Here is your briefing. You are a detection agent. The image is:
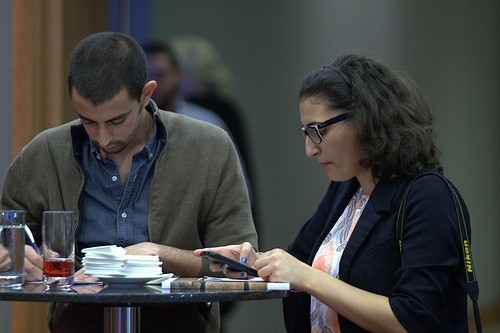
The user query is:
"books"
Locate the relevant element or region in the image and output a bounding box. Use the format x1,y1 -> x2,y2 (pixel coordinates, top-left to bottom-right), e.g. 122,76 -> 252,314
162,278 -> 291,291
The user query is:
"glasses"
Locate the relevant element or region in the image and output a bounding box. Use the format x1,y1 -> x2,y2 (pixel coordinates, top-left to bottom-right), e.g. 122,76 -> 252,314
298,111 -> 355,145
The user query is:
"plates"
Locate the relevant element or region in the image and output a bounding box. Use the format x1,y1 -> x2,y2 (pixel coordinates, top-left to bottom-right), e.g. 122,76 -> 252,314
95,277 -> 154,287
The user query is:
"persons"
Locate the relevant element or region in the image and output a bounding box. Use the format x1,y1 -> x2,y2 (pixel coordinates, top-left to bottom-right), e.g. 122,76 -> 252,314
0,31 -> 259,332
193,53 -> 472,333
137,37 -> 252,206
168,33 -> 251,180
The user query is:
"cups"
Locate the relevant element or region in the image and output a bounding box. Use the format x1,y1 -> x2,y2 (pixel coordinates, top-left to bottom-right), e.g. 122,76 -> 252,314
0,210 -> 26,287
42,210 -> 76,287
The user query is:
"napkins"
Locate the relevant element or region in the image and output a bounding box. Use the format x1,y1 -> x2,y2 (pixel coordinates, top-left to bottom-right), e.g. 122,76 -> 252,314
81,245 -> 174,278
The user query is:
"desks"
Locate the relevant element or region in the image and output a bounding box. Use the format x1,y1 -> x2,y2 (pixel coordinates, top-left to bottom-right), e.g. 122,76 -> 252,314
0,278 -> 287,333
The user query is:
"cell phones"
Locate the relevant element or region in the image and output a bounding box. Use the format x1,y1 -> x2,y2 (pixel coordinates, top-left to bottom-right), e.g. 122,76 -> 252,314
200,251 -> 259,277
